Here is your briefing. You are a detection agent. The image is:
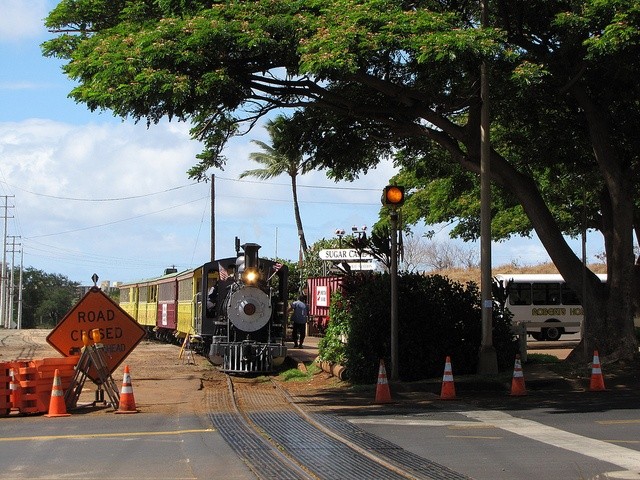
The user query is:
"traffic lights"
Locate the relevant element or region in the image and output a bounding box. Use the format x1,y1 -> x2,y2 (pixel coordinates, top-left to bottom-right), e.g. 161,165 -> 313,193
381,184 -> 406,208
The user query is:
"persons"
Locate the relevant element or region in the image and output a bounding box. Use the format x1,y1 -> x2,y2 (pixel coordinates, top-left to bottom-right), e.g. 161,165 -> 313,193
207,281 -> 218,312
287,295 -> 308,349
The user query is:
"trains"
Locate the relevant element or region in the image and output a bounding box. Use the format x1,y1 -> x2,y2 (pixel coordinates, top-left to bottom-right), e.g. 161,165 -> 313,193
307,276 -> 343,337
118,243 -> 291,377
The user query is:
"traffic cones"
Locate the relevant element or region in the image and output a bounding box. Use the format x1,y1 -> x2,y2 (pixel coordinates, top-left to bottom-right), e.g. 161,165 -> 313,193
584,351 -> 609,392
370,358 -> 399,404
113,365 -> 139,414
505,357 -> 532,397
43,368 -> 71,418
434,356 -> 460,401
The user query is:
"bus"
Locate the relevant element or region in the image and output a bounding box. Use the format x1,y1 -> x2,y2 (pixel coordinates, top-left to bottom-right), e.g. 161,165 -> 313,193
493,274 -> 609,341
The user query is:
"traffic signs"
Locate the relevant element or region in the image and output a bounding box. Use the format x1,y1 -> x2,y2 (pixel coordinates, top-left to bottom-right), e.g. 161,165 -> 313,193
46,286 -> 147,388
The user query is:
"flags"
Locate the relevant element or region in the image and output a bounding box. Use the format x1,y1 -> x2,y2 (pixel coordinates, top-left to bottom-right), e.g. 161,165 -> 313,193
219,264 -> 228,282
272,261 -> 281,271
